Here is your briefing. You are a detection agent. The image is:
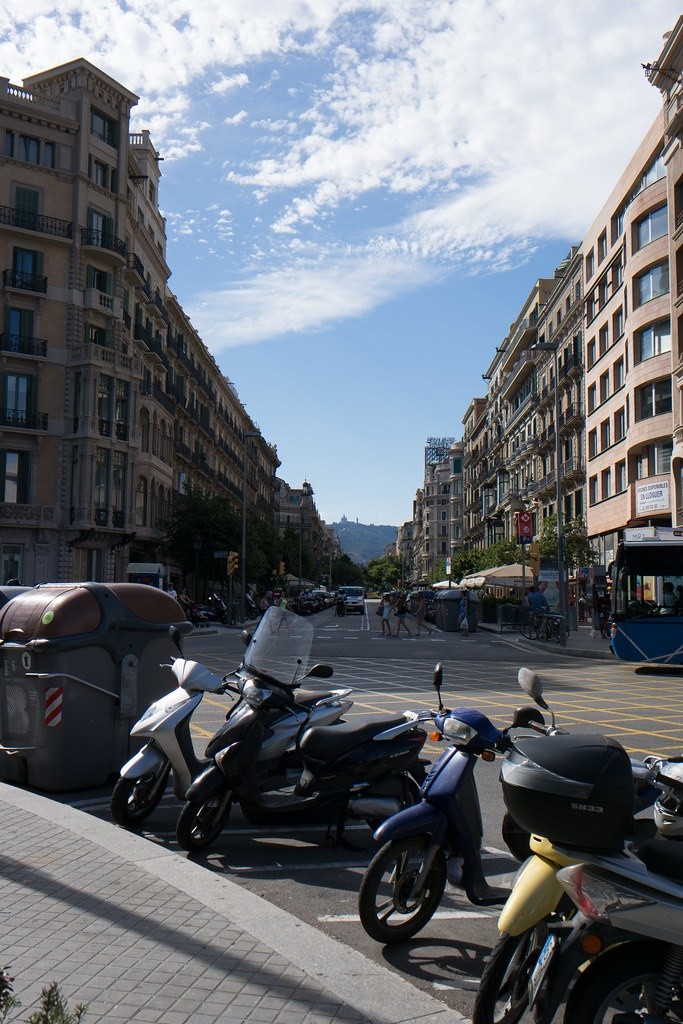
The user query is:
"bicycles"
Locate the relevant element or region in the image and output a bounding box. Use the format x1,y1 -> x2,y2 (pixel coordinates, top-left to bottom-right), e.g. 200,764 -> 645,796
519,605 -> 570,645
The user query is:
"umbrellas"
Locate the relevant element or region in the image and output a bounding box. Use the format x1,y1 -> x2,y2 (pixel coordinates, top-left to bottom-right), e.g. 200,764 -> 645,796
433,579 -> 458,588
465,563 -> 533,578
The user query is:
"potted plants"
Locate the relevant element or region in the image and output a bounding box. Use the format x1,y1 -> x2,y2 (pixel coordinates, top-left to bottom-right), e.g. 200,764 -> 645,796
480,597 -> 528,625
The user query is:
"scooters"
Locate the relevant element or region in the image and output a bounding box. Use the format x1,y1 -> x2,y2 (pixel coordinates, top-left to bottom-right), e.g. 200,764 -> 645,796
287,594 -> 339,616
176,605 -> 437,852
110,625 -> 354,828
358,662 -> 546,944
467,666 -> 683,1024
197,592 -> 258,625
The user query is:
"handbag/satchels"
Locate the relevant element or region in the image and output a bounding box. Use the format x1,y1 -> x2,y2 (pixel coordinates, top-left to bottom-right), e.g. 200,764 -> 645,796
459,617 -> 468,629
376,603 -> 384,616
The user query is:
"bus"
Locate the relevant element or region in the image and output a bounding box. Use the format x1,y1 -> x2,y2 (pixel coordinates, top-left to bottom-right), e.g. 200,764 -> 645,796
608,526 -> 683,665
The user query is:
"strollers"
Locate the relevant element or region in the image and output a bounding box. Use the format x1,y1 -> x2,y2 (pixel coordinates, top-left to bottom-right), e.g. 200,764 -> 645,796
189,602 -> 210,628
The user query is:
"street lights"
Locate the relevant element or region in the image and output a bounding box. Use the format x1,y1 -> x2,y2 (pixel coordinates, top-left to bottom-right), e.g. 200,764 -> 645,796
298,506 -> 309,594
239,430 -> 261,623
329,552 -> 335,592
530,341 -> 568,648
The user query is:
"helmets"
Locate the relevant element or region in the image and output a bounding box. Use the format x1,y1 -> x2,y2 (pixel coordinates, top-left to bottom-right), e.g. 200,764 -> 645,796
653,762 -> 683,836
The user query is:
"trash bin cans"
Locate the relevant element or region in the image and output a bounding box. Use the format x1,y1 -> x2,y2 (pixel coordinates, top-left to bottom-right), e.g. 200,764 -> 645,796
0,582 -> 194,794
434,590 -> 479,632
410,591 -> 431,617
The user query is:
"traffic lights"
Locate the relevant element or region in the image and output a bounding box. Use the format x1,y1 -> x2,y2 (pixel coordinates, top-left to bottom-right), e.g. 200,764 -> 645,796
227,556 -> 235,575
279,562 -> 286,576
229,551 -> 239,569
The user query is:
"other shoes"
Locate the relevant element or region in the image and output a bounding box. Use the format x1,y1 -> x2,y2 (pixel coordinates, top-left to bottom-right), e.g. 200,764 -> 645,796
387,634 -> 392,636
415,633 -> 420,636
429,628 -> 432,635
381,634 -> 385,636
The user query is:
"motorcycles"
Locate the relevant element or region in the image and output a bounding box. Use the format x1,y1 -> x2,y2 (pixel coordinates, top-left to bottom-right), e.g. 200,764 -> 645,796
332,600 -> 346,617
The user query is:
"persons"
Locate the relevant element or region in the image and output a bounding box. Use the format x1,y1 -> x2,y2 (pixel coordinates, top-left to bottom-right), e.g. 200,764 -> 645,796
193,605 -> 205,618
527,585 -> 550,638
179,587 -> 195,617
297,590 -> 312,599
166,583 -> 177,601
568,591 -> 588,622
381,594 -> 412,638
279,591 -> 293,611
414,591 -> 432,636
659,582 -> 679,605
335,590 -> 347,617
260,591 -> 273,616
457,590 -> 469,636
246,590 -> 254,607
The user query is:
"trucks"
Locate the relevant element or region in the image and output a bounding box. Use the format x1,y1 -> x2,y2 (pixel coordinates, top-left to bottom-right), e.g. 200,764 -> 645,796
337,586 -> 367,615
